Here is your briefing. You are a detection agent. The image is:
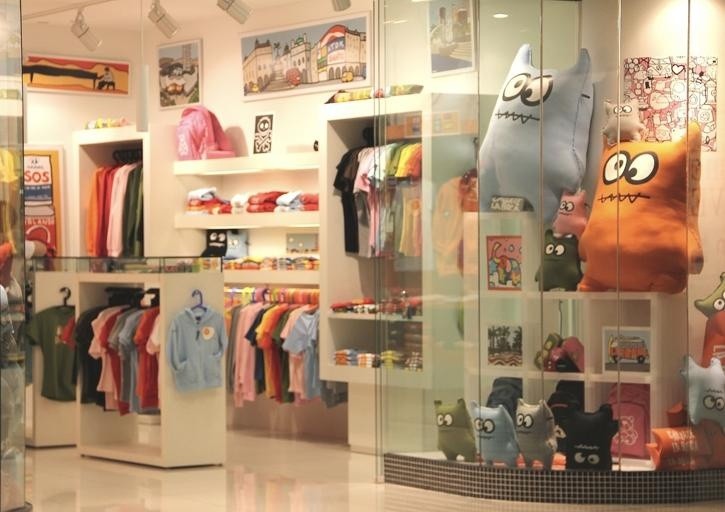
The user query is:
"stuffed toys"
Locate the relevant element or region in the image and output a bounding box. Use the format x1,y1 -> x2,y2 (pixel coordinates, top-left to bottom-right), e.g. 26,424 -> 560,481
680,357 -> 722,428
552,404 -> 618,471
476,43 -> 592,226
603,98 -> 642,142
474,402 -> 519,468
694,273 -> 725,369
514,398 -> 555,471
534,231 -> 583,291
554,188 -> 588,240
436,399 -> 475,463
578,123 -> 702,293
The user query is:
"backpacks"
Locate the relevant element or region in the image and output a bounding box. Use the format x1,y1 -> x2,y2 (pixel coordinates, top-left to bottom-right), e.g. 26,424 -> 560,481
177,105 -> 235,160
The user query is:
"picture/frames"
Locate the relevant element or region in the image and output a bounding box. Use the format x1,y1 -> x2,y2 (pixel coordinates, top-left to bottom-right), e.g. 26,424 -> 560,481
240,13 -> 372,103
486,234 -> 523,291
156,37 -> 204,111
426,0 -> 477,77
601,325 -> 652,375
24,55 -> 132,98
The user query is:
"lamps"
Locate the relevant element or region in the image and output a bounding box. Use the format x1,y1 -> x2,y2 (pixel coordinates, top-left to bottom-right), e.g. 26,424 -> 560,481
72,6 -> 104,52
332,0 -> 351,12
215,0 -> 254,24
147,0 -> 181,39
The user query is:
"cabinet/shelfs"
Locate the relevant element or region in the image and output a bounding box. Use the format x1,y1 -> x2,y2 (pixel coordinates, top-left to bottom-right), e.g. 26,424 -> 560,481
172,146 -> 322,287
70,129 -> 150,276
460,211 -> 688,459
76,274 -> 225,470
24,270 -> 77,450
319,89 -> 480,388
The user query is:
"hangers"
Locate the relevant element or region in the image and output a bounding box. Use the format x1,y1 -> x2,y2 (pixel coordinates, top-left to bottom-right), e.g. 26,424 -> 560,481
53,286 -> 78,316
222,283 -> 317,304
88,287 -> 161,313
190,287 -> 210,316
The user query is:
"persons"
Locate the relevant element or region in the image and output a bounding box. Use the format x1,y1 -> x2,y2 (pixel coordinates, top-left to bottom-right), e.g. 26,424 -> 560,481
97,64 -> 115,91
